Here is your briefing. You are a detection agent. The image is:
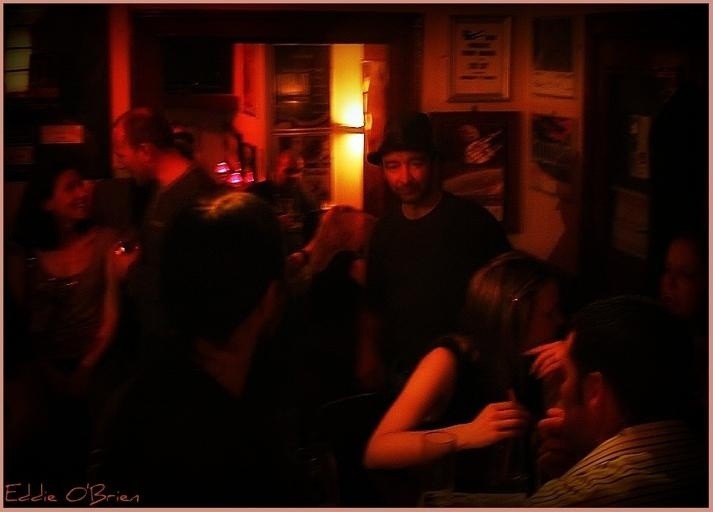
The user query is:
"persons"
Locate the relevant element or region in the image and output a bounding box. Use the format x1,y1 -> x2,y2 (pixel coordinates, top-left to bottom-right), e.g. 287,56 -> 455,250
4,109 -> 708,507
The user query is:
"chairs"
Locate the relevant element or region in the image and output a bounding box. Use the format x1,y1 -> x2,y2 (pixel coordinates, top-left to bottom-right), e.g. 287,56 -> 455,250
447,12 -> 579,101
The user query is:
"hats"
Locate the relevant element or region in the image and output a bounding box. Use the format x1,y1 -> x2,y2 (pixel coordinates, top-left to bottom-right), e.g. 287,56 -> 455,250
367,112 -> 437,165
